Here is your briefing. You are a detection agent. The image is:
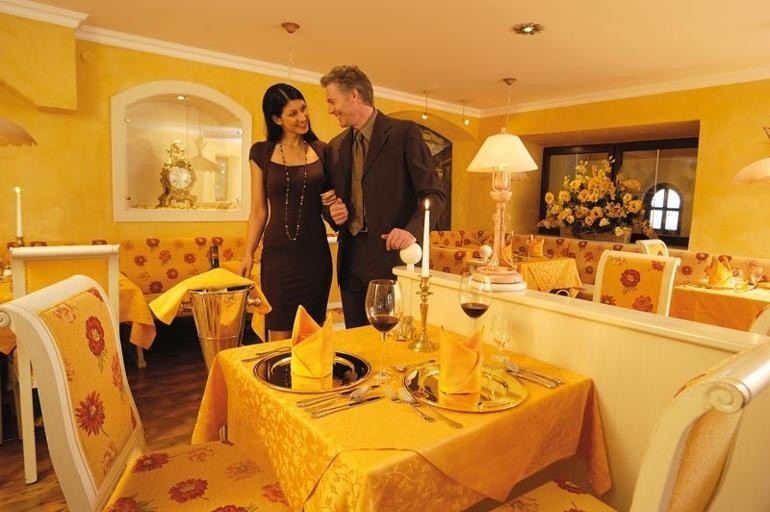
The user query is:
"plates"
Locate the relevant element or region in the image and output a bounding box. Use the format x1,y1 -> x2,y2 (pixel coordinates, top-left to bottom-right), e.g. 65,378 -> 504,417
402,365 -> 530,414
690,279 -> 770,290
253,350 -> 374,395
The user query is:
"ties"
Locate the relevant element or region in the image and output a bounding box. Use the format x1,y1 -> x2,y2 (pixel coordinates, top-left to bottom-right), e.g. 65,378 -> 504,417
345,130 -> 365,238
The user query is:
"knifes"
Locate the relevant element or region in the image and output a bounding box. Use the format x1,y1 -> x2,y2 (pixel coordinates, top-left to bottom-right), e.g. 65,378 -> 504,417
297,385 -> 384,418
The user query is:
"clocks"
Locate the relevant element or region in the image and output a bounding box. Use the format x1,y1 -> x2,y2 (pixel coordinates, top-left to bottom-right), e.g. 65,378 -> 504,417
157,140 -> 200,209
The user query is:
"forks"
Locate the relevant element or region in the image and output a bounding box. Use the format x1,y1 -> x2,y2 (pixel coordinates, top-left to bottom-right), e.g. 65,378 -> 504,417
503,359 -> 566,389
383,385 -> 462,429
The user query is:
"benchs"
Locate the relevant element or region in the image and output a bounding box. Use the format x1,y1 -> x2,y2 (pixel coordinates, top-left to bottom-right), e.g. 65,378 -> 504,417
427,229 -> 770,326
0,234 -> 261,322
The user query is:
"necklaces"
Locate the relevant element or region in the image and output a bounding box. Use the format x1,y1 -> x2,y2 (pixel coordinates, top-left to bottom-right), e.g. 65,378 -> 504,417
278,140 -> 308,240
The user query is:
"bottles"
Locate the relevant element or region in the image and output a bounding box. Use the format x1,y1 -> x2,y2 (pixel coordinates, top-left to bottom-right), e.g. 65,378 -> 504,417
205,244 -> 230,293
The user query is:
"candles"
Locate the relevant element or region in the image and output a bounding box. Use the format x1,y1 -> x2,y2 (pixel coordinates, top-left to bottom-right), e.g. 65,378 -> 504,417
15,186 -> 24,237
420,198 -> 433,277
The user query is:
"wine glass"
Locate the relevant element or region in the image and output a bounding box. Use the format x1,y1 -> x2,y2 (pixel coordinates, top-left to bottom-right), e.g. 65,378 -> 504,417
362,280 -> 404,383
456,270 -> 494,368
492,319 -> 513,362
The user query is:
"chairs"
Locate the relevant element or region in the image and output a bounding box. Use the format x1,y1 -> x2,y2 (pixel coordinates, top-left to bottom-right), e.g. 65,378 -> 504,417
487,333 -> 770,512
0,273 -> 304,512
8,244 -> 120,486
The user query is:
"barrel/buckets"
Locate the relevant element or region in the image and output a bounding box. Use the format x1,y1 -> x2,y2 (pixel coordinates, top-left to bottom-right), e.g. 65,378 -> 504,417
178,284 -> 263,376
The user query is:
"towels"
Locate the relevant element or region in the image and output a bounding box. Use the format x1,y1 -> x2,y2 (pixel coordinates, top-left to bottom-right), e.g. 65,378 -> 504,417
287,304 -> 334,378
440,393 -> 483,410
292,375 -> 332,394
438,325 -> 485,396
146,266 -> 271,344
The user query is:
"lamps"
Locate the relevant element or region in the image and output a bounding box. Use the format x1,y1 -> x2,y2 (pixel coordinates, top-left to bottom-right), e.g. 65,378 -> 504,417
0,115 -> 39,150
465,125 -> 539,292
178,100 -> 221,175
460,99 -> 472,126
421,89 -> 431,120
479,74 -> 531,185
734,155 -> 770,185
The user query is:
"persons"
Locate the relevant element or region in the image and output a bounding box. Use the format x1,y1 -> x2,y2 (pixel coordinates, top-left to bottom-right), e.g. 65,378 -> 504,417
320,63 -> 448,332
237,82 -> 336,342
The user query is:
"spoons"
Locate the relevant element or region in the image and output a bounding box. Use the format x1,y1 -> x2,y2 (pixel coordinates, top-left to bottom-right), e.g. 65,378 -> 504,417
390,358 -> 438,373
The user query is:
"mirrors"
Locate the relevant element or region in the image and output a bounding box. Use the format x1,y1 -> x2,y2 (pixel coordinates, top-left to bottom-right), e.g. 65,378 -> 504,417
106,77 -> 254,224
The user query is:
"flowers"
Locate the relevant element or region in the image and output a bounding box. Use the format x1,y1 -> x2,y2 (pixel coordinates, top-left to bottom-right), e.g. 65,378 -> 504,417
528,151 -> 663,241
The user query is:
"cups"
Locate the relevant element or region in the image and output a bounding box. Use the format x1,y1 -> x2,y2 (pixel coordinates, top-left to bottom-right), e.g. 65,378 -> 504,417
750,269 -> 763,283
